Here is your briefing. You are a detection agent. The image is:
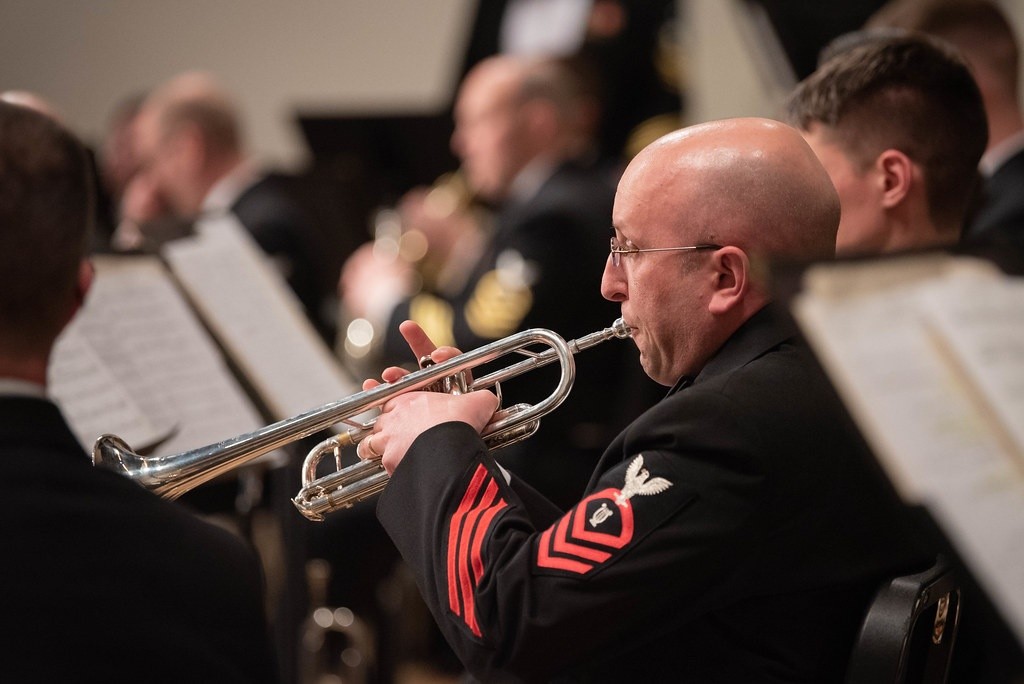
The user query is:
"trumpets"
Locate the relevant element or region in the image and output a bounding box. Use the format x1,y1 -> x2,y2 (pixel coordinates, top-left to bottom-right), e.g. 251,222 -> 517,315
89,308 -> 631,524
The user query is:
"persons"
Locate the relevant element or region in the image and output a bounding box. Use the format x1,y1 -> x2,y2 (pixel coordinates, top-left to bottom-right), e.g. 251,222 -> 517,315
357,117 -> 891,684
0,0 -> 1023,683
1,98 -> 284,684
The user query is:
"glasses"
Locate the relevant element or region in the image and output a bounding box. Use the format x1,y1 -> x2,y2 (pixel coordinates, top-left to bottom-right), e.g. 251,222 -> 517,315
609,232 -> 722,267
